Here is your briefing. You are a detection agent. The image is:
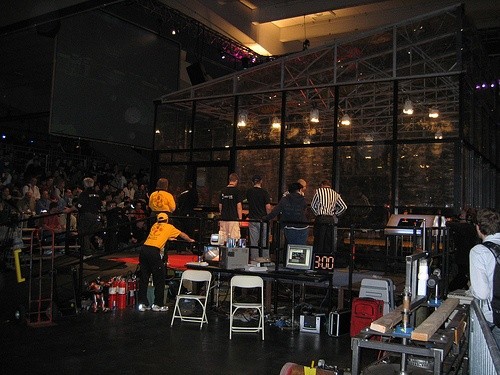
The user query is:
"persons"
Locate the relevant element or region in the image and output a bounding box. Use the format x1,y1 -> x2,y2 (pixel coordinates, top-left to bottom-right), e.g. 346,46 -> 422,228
138,212 -> 195,311
0,153 -> 149,246
179,182 -> 198,216
218,173 -> 243,239
261,182 -> 310,245
246,175 -> 270,259
348,185 -> 372,229
63,177 -> 99,260
465,208 -> 500,375
149,178 -> 176,225
283,179 -> 306,197
310,179 -> 347,253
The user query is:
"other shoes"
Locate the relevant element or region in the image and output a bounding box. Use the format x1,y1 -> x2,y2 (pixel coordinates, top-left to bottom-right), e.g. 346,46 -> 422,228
138,304 -> 151,312
151,305 -> 169,312
83,255 -> 92,260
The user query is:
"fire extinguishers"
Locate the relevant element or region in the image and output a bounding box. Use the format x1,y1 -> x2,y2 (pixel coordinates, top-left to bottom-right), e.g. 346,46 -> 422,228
89,274 -> 137,313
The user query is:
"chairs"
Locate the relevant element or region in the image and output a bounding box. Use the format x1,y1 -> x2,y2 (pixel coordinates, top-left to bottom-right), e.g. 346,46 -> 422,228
229,276 -> 265,341
170,269 -> 212,330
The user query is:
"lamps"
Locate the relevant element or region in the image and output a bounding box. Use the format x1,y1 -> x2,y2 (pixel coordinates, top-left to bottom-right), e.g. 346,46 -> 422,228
309,103 -> 319,123
272,116 -> 281,129
237,112 -> 248,127
428,105 -> 439,117
402,94 -> 414,114
341,113 -> 351,126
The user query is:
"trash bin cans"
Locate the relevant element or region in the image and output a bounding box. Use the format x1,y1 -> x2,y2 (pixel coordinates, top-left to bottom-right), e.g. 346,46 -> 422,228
146,284 -> 169,306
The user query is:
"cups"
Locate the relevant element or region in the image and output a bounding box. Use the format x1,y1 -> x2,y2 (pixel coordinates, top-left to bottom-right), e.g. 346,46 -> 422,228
238,239 -> 246,248
226,238 -> 236,248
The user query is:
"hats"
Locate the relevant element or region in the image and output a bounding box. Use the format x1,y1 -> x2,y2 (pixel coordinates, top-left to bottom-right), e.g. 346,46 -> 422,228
252,175 -> 263,181
157,212 -> 169,222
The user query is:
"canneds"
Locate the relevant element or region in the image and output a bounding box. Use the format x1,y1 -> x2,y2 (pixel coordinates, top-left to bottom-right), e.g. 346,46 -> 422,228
230,238 -> 236,248
238,239 -> 246,248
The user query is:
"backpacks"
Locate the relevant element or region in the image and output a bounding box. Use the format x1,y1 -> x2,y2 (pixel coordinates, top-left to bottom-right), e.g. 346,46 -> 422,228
480,240 -> 500,329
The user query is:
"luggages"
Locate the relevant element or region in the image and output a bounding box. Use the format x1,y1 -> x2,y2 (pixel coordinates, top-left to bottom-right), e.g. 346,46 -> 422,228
350,296 -> 385,341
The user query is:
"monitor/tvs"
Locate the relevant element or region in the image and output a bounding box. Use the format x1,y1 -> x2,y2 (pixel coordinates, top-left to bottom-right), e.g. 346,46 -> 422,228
286,244 -> 313,270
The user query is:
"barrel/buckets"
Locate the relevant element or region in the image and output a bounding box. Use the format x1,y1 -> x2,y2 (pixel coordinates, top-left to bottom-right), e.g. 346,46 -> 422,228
146,285 -> 169,305
